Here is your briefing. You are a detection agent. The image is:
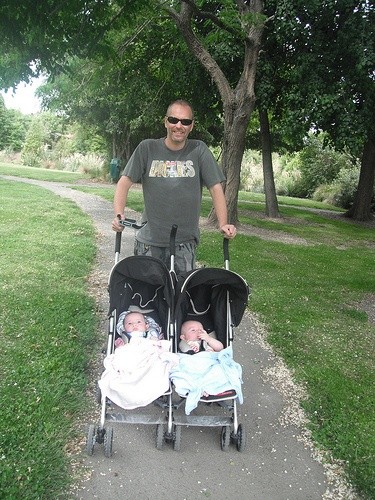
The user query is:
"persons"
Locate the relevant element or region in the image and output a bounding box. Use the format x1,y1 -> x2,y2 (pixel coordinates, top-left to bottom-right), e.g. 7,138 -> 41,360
112,100 -> 237,274
100,312 -> 171,409
180,320 -> 235,397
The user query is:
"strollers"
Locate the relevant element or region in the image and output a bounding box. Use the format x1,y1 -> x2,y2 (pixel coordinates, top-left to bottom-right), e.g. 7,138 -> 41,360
87,214 -> 250,459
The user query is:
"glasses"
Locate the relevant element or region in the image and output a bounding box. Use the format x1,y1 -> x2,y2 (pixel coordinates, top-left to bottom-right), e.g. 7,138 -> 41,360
167,117 -> 193,126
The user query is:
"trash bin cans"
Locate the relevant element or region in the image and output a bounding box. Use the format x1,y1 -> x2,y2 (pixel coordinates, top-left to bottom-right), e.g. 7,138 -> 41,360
110,158 -> 122,183
